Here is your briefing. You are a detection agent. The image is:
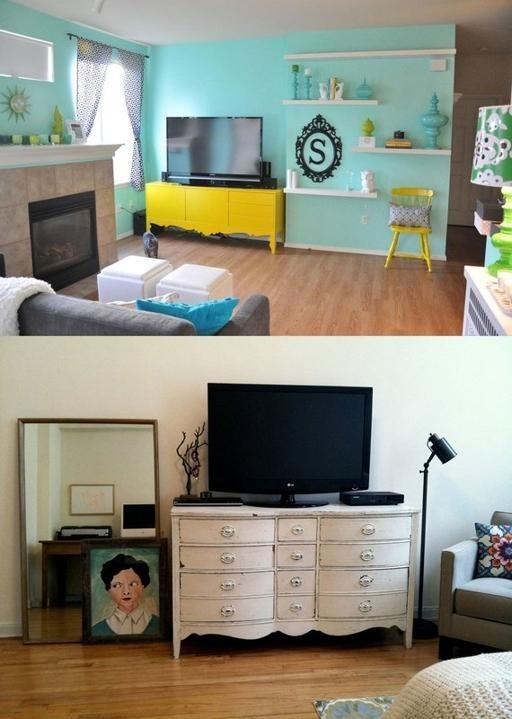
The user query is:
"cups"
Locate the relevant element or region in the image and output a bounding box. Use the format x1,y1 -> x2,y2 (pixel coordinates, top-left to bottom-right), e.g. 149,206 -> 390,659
497,270 -> 512,289
0,134 -> 72,145
504,280 -> 512,297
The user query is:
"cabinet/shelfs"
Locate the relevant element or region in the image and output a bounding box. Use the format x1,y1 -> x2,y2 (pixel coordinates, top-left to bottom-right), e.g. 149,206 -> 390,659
169,503 -> 420,660
282,49 -> 452,198
144,180 -> 285,254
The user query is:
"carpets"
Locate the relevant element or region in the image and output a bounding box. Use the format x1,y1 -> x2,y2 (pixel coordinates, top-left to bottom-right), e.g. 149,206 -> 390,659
310,694 -> 398,719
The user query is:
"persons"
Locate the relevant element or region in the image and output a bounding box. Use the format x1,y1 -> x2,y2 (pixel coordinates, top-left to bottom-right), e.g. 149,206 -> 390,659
90,553 -> 160,639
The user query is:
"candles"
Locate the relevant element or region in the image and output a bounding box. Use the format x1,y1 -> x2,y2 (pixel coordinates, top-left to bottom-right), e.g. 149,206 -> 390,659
304,68 -> 311,75
286,170 -> 298,189
292,65 -> 298,72
0,134 -> 72,146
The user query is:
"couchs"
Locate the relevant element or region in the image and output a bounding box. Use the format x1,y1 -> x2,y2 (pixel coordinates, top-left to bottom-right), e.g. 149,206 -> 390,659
0,277 -> 270,336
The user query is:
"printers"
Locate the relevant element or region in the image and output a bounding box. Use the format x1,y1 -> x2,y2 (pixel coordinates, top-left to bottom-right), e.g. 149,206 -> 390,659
60,525 -> 112,540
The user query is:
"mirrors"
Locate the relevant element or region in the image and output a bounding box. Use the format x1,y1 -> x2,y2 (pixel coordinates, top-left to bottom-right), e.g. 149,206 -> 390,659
17,418 -> 161,645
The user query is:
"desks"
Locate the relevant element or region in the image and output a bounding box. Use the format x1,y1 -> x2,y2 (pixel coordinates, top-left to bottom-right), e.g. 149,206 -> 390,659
462,266 -> 512,336
39,540 -> 82,609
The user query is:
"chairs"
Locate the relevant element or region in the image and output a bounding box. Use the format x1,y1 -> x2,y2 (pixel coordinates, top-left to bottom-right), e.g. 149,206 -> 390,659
384,188 -> 434,273
438,511 -> 512,660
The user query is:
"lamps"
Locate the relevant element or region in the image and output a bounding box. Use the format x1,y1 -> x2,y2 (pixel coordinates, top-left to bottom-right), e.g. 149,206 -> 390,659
470,105 -> 512,277
412,433 -> 458,639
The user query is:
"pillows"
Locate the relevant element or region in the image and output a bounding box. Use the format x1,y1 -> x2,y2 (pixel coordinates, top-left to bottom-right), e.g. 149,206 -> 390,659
136,298 -> 240,336
472,522 -> 512,579
105,292 -> 179,309
388,201 -> 432,228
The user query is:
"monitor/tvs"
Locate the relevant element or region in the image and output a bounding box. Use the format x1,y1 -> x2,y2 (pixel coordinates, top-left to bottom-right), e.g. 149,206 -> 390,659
207,383 -> 374,508
166,116 -> 263,187
119,503 -> 156,539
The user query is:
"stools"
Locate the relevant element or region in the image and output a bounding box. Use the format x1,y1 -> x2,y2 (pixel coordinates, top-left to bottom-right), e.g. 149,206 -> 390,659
97,254 -> 173,304
156,263 -> 233,305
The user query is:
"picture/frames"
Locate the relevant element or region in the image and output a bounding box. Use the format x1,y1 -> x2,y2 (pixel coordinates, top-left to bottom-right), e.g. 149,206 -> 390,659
69,483 -> 115,515
81,537 -> 172,645
